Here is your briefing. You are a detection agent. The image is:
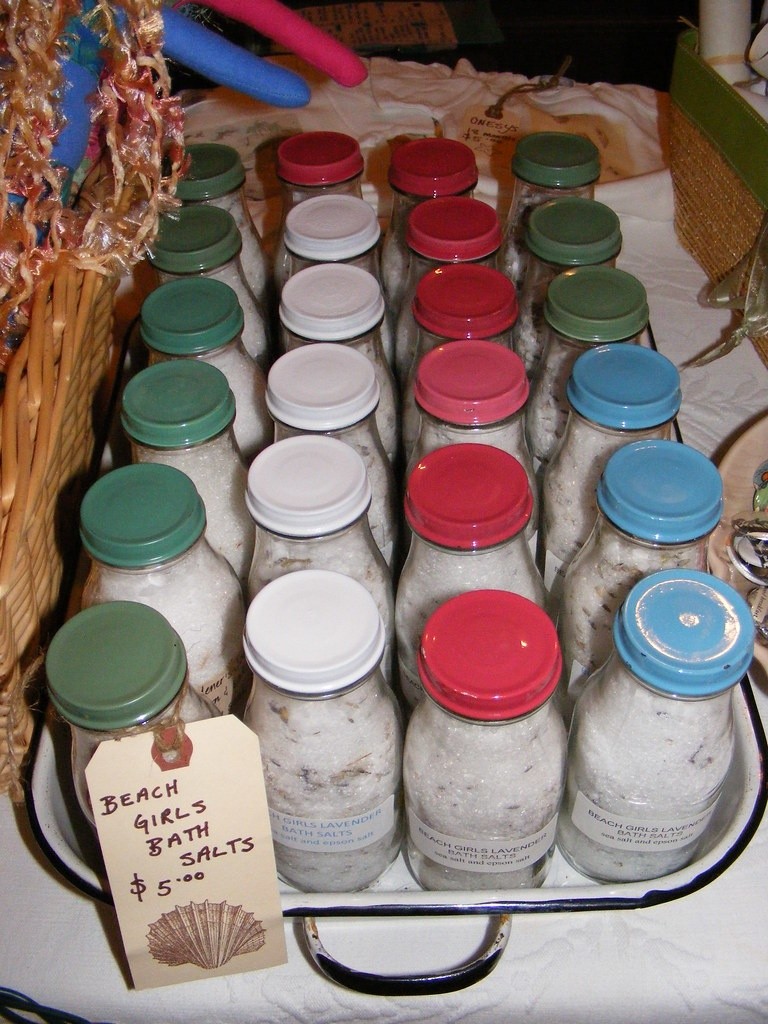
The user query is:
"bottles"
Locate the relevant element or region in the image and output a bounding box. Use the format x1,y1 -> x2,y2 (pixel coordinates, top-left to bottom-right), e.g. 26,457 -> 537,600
44,127 -> 753,891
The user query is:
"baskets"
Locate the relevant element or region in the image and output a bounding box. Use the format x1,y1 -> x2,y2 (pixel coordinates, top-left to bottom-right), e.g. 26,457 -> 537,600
667,23 -> 768,369
0,3 -> 192,807
